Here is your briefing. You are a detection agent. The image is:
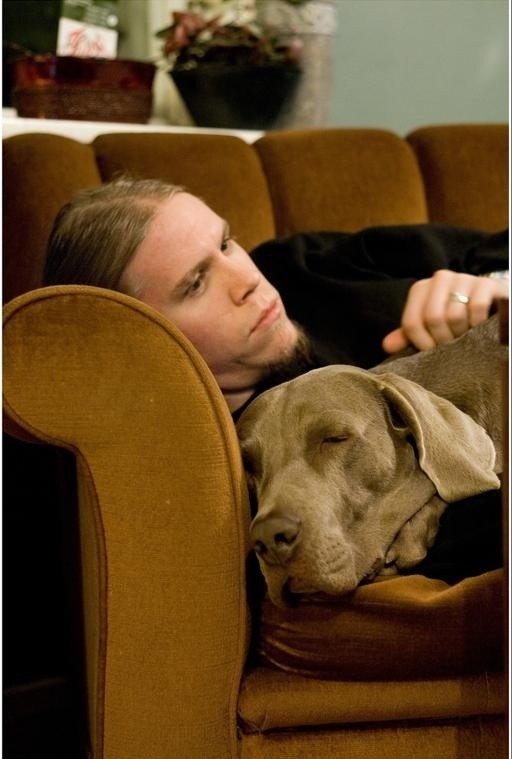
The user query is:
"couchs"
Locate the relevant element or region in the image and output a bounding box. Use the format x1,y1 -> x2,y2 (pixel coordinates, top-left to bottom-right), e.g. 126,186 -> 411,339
2,124 -> 509,759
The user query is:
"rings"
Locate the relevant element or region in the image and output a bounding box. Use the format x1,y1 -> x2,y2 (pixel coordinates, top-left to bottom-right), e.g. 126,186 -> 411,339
448,290 -> 469,307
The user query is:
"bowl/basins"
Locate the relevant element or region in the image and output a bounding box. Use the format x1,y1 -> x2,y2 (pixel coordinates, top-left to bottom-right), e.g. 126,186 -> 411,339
13,53 -> 159,124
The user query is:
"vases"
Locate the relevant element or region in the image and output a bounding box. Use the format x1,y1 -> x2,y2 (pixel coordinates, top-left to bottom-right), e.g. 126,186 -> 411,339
168,68 -> 301,130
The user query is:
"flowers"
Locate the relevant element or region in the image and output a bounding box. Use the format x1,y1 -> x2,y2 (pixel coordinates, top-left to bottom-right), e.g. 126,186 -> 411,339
155,0 -> 296,69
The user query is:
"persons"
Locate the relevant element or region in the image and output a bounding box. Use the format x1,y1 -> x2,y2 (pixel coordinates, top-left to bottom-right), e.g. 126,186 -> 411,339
38,173 -> 508,586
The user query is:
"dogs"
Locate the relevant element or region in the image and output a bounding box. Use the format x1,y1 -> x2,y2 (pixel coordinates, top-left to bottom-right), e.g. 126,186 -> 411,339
234,309 -> 509,612
